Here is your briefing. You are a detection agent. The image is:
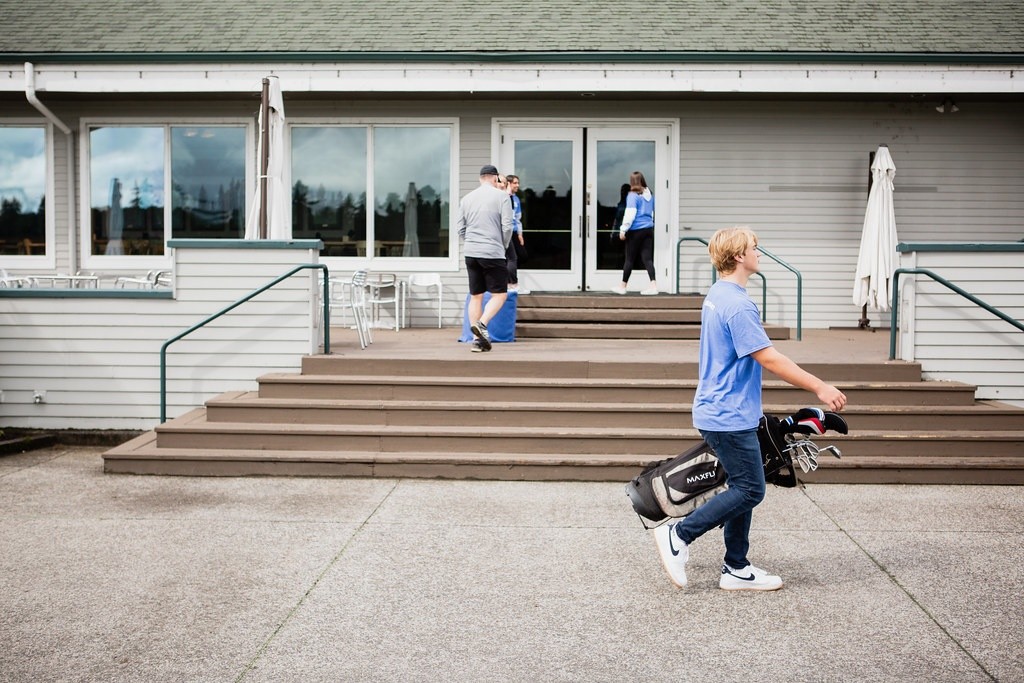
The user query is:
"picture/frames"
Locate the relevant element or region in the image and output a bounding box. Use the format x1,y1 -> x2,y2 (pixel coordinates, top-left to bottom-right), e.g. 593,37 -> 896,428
191,185 -> 224,231
124,209 -> 149,230
292,201 -> 303,231
151,207 -> 186,231
308,203 -> 343,231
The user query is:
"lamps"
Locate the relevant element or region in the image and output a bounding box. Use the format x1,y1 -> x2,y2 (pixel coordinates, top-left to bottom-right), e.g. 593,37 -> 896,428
950,100 -> 960,113
936,100 -> 948,113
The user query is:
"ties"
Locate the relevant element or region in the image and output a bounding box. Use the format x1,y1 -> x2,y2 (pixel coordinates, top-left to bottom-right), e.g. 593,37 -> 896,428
510,195 -> 515,209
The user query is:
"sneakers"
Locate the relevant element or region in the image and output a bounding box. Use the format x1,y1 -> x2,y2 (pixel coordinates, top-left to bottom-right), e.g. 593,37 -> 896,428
470,321 -> 492,349
471,339 -> 483,351
653,521 -> 689,587
719,562 -> 782,591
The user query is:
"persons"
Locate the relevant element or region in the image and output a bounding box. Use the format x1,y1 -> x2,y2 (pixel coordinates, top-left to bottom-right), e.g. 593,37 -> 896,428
457,165 -> 514,352
497,173 -> 508,190
611,171 -> 658,295
505,174 -> 532,295
610,183 -> 631,239
653,227 -> 847,591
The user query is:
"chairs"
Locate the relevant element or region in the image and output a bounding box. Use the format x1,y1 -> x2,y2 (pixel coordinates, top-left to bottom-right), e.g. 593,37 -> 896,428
319,270 -> 443,351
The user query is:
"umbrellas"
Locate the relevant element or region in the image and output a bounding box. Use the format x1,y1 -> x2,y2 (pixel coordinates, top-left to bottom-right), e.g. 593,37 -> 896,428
104,177 -> 125,255
852,144 -> 901,319
402,182 -> 420,256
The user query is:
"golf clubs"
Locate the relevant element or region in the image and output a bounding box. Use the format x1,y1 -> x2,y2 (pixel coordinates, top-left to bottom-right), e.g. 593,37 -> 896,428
782,433 -> 842,473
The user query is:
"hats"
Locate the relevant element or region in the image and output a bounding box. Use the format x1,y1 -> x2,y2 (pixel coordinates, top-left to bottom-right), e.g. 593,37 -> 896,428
480,164 -> 501,182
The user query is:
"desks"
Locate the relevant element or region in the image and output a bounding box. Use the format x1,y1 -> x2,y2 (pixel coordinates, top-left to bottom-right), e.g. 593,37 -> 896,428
351,279 -> 394,330
325,241 -> 411,256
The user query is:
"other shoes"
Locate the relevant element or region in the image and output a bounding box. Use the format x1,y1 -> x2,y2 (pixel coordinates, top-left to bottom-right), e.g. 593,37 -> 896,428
640,286 -> 659,295
611,286 -> 627,295
